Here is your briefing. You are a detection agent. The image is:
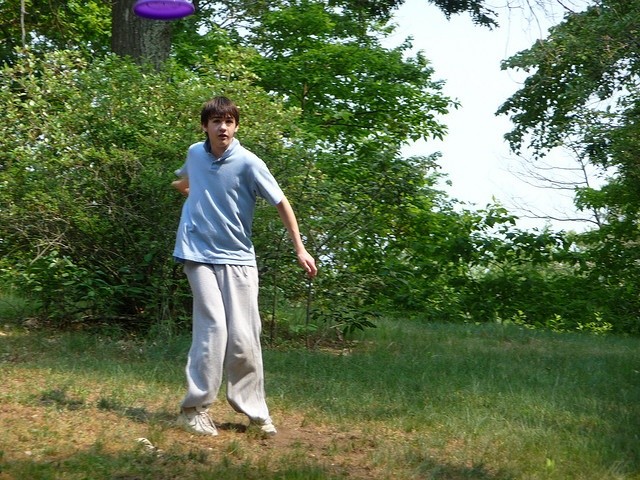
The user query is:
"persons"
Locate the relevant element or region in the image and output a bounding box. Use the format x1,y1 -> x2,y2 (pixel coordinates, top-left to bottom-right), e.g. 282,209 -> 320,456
170,94 -> 319,437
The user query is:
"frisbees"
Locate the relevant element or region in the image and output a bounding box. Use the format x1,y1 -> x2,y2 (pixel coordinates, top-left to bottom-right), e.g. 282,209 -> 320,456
133,0 -> 195,19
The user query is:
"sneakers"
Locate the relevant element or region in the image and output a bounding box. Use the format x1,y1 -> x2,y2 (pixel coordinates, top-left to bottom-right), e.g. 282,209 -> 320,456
245,420 -> 278,437
177,402 -> 218,436
